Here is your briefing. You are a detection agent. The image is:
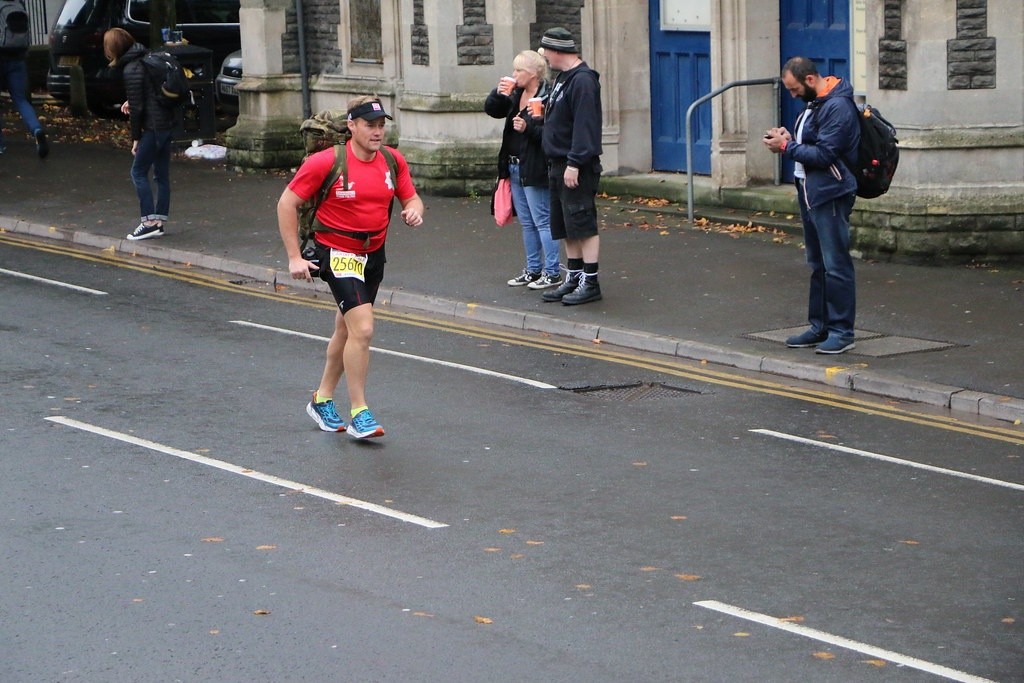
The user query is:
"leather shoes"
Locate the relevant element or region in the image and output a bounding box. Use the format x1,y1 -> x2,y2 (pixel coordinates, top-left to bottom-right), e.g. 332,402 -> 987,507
561,271 -> 602,304
541,263 -> 584,302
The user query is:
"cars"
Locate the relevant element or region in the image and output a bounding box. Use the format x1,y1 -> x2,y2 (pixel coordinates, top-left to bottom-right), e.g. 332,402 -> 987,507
215,47 -> 242,115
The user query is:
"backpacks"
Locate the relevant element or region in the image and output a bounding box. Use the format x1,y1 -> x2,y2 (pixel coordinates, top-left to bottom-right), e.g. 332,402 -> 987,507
299,108 -> 400,240
0,0 -> 29,53
138,48 -> 191,113
813,96 -> 900,200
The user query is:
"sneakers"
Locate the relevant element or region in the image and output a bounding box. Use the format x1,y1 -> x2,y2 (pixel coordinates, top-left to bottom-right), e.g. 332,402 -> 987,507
126,223 -> 160,241
785,329 -> 829,347
155,225 -> 164,235
527,271 -> 562,289
346,409 -> 385,438
306,392 -> 347,432
507,269 -> 542,285
815,334 -> 856,354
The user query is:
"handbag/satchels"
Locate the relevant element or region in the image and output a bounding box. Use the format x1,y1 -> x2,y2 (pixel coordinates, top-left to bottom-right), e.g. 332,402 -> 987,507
491,183 -> 517,217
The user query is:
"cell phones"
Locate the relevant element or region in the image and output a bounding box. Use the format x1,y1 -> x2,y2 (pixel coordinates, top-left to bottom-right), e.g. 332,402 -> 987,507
764,135 -> 772,140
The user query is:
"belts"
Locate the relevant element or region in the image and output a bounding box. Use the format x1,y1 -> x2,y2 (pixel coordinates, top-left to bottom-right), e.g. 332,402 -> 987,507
508,154 -> 521,166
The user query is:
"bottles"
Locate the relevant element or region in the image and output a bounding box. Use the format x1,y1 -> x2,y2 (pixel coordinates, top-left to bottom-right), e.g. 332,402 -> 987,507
869,159 -> 879,179
301,247 -> 317,277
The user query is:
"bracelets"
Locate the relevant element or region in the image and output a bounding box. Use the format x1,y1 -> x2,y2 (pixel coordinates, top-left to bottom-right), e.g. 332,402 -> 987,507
780,140 -> 788,152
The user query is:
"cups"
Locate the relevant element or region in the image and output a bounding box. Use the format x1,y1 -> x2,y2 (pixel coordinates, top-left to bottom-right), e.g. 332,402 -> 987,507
501,77 -> 516,97
172,31 -> 182,43
528,97 -> 542,117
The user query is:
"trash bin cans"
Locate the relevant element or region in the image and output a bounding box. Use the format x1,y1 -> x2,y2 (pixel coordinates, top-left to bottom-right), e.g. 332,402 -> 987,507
152,46 -> 216,152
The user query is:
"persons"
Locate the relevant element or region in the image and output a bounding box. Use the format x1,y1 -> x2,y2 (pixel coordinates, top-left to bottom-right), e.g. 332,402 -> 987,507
1,0 -> 49,159
762,57 -> 862,354
104,27 -> 180,241
526,27 -> 604,305
277,96 -> 425,438
484,48 -> 563,289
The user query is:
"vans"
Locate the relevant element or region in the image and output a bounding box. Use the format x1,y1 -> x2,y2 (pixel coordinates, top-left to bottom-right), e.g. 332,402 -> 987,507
45,0 -> 243,114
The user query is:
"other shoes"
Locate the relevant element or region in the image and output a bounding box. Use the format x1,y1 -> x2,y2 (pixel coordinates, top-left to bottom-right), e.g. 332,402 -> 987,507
35,129 -> 49,158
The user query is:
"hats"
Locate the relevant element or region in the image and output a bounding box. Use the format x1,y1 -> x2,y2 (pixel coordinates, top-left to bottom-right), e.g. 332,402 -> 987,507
540,27 -> 578,53
348,100 -> 393,122
